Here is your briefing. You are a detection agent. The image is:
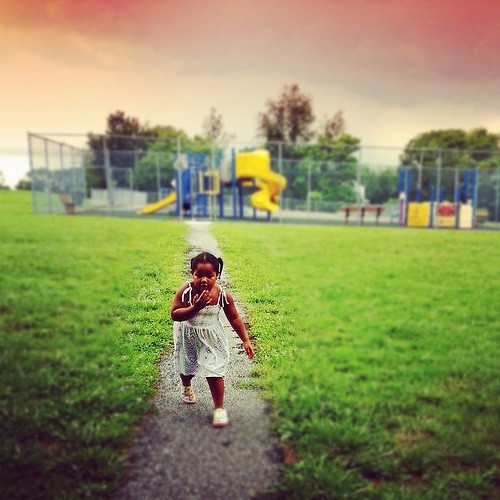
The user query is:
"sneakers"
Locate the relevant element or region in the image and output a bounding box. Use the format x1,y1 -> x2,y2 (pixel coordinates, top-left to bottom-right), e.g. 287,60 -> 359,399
212,407 -> 228,427
180,386 -> 197,404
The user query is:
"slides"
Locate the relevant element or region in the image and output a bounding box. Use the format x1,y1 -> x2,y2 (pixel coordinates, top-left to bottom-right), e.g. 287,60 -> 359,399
136,191 -> 177,215
235,150 -> 288,213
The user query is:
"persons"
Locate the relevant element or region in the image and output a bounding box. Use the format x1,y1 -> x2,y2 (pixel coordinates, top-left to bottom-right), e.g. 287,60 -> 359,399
171,252 -> 255,428
